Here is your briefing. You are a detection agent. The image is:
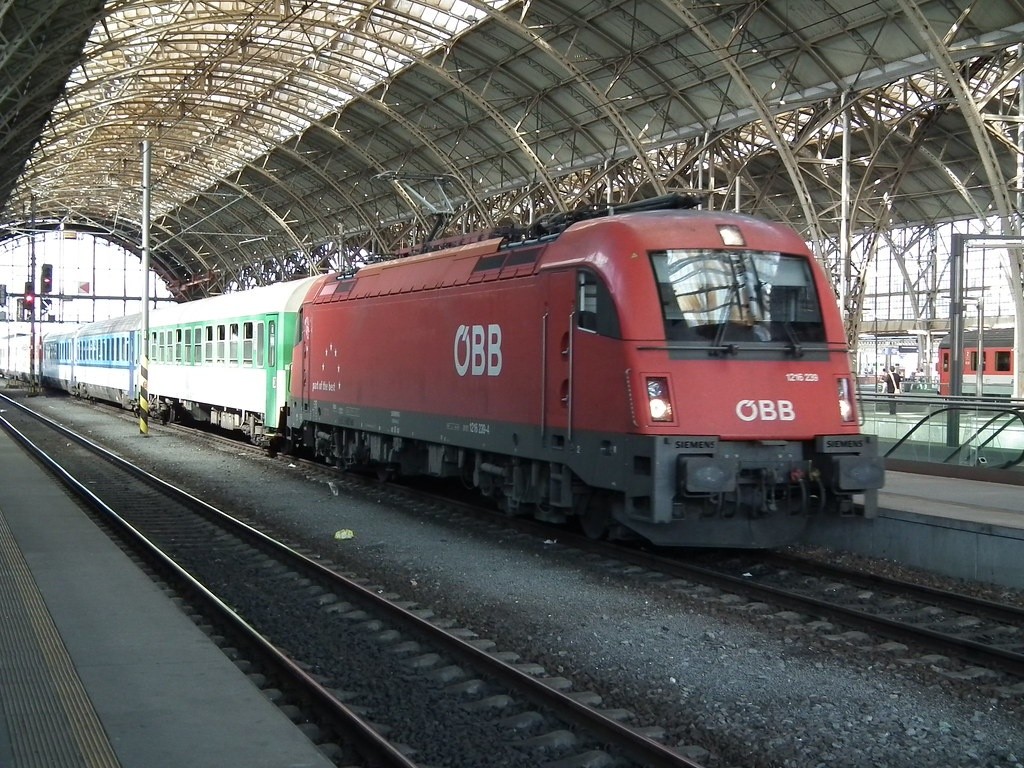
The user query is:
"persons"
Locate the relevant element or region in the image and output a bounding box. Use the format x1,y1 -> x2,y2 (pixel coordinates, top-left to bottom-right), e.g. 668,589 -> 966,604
864,362 -> 924,394
881,366 -> 901,415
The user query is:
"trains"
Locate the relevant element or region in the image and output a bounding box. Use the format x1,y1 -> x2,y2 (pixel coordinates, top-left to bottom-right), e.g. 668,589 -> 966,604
0,190 -> 886,550
938,327 -> 1015,406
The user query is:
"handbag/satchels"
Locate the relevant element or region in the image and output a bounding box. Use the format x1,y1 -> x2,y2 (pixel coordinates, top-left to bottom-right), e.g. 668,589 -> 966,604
894,389 -> 900,398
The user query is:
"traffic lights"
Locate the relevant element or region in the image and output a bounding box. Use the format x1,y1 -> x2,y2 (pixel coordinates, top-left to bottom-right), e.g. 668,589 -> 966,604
23,281 -> 33,309
41,264 -> 52,291
